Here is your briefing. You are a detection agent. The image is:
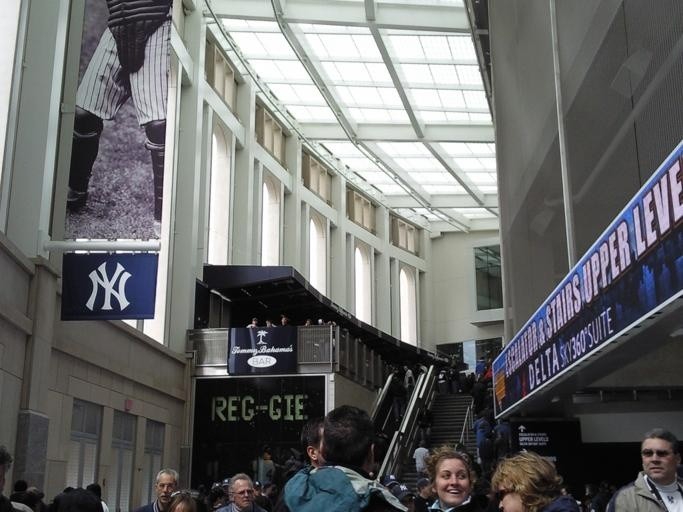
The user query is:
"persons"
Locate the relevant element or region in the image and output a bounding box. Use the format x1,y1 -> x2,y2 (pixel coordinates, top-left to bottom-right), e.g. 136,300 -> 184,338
394,362 -> 428,400
388,377 -> 409,422
438,346 -> 493,404
66,0 -> 173,237
247,314 -> 334,327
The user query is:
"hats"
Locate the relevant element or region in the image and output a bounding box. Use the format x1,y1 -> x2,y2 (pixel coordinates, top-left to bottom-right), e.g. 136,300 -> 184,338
211,478 -> 262,489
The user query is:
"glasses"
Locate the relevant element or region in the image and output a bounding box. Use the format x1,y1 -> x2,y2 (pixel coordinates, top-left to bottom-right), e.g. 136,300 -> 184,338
641,448 -> 675,457
232,490 -> 253,495
